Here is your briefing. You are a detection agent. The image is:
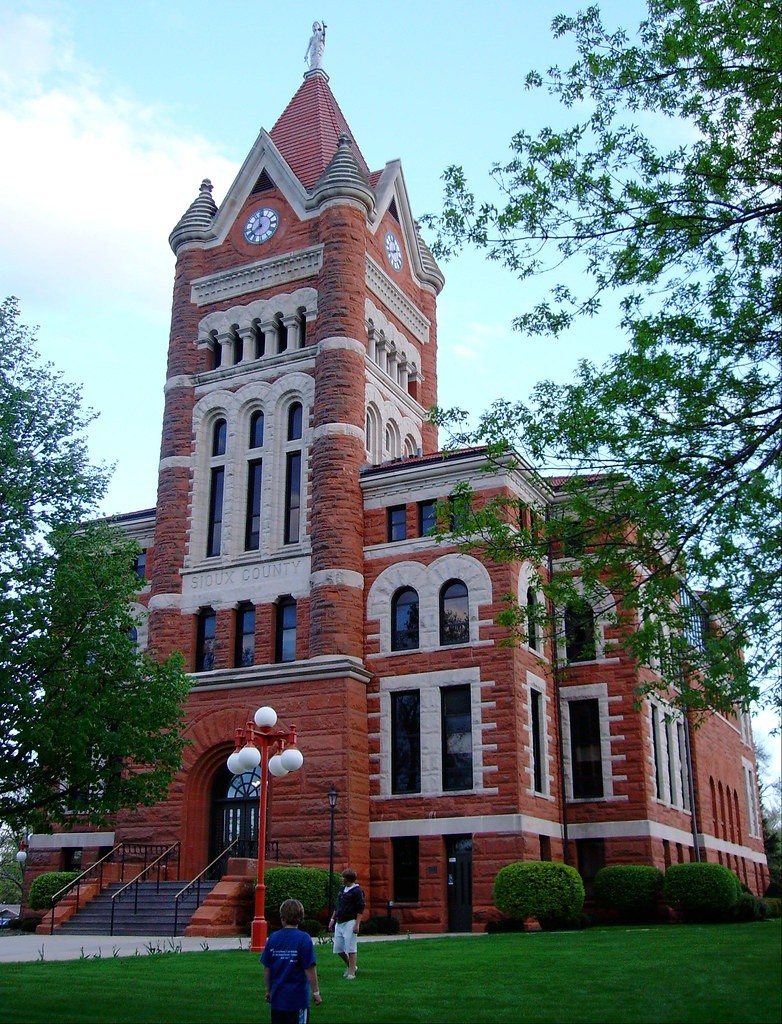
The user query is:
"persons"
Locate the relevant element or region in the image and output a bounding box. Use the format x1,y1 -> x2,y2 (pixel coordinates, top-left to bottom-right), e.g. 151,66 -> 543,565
259,900 -> 322,1024
328,868 -> 365,980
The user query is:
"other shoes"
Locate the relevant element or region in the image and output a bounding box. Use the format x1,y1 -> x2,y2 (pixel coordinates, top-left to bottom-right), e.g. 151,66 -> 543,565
348,974 -> 356,979
343,966 -> 358,977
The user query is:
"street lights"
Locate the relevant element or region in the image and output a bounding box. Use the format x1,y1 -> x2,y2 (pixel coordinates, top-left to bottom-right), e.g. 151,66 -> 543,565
226,707 -> 303,952
324,788 -> 340,933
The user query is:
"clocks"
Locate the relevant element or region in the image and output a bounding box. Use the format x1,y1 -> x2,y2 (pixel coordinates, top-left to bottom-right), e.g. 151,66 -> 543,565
385,231 -> 403,272
243,207 -> 279,245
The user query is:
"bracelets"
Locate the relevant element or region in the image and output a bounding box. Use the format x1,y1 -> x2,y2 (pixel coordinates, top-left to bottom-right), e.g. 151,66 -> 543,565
313,991 -> 319,996
